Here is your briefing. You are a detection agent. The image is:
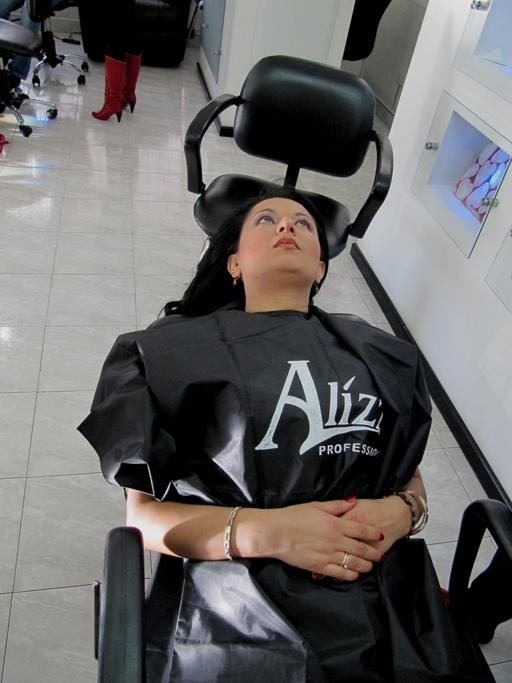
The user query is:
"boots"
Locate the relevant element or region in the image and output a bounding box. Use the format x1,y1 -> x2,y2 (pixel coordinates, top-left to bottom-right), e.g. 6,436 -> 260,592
122,50 -> 142,114
91,53 -> 123,123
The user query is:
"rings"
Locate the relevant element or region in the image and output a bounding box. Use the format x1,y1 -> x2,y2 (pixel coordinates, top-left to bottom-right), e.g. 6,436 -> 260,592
339,550 -> 354,569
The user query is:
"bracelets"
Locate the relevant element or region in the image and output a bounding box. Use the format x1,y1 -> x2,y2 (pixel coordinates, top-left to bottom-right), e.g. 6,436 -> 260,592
400,484 -> 431,536
393,488 -> 418,539
222,505 -> 243,561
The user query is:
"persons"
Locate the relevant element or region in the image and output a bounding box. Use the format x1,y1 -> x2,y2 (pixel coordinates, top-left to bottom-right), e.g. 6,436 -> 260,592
0,0 -> 50,94
76,188 -> 490,680
86,0 -> 149,123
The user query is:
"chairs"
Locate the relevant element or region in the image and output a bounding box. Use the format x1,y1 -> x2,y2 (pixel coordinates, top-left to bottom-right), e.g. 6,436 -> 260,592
89,497 -> 511,683
178,52 -> 399,262
0,0 -> 83,136
